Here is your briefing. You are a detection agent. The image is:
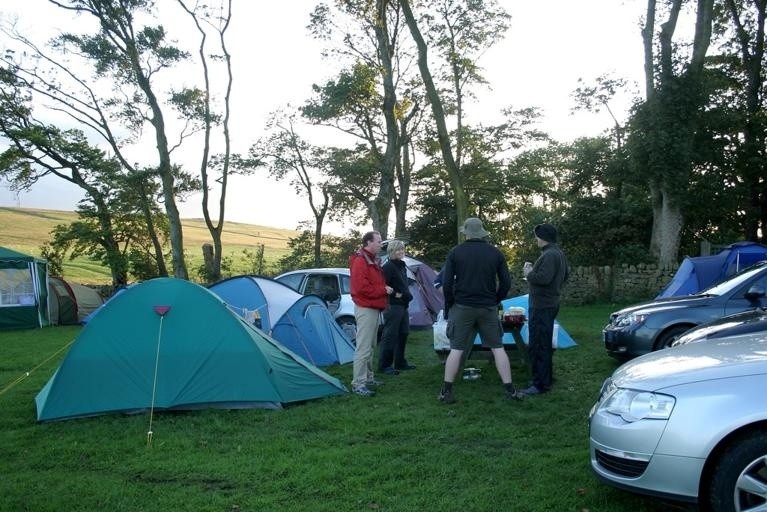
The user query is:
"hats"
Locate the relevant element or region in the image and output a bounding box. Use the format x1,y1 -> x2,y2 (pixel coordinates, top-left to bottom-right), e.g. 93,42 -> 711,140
459,218 -> 490,238
534,224 -> 557,243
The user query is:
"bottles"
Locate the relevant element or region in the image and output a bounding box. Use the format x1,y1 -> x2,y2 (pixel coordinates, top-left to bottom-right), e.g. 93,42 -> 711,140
464,367 -> 481,377
436,310 -> 443,325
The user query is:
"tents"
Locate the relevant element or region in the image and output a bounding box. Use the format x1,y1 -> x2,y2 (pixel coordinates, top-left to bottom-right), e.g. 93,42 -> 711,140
400,254 -> 445,330
35,275 -> 350,423
46,276 -> 106,327
0,246 -> 51,331
207,274 -> 356,369
653,239 -> 767,301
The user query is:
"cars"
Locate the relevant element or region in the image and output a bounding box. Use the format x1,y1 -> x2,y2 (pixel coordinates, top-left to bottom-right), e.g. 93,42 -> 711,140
272,268 -> 385,347
602,259 -> 767,360
587,334 -> 767,512
672,307 -> 767,346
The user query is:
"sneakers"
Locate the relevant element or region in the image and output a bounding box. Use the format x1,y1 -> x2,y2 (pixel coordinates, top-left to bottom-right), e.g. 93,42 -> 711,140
367,380 -> 385,386
526,386 -> 539,394
438,392 -> 454,403
352,387 -> 374,397
397,362 -> 417,369
505,390 -> 525,400
382,368 -> 399,375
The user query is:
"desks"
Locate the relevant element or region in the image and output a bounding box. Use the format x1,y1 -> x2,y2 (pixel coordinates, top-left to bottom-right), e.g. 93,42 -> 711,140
463,318 -> 528,366
0,304 -> 37,329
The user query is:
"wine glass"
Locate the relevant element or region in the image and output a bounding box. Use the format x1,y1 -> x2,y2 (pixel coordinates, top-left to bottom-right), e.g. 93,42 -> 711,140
522,262 -> 532,280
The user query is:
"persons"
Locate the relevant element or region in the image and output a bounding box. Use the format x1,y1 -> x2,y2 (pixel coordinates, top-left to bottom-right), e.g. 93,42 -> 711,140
377,239 -> 416,377
434,217 -> 524,405
519,221 -> 571,395
433,264 -> 444,296
348,231 -> 393,397
